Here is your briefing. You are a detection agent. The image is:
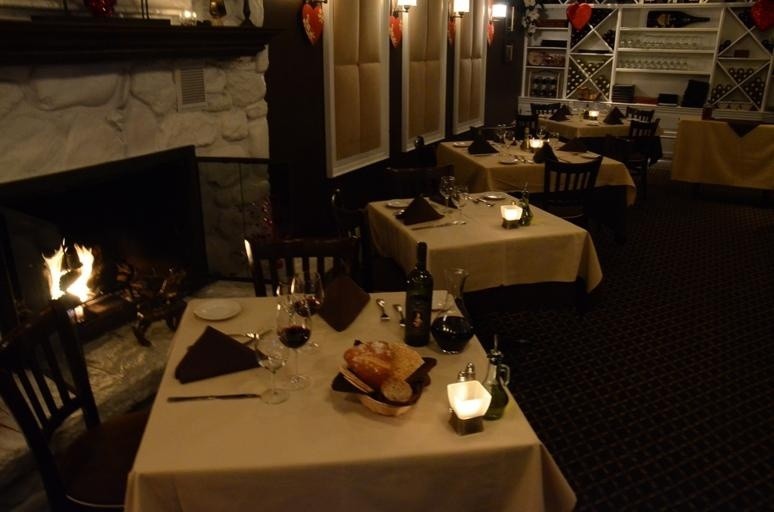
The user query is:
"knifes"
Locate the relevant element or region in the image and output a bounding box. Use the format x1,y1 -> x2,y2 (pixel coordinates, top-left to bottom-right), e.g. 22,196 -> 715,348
412,221 -> 465,231
168,394 -> 260,402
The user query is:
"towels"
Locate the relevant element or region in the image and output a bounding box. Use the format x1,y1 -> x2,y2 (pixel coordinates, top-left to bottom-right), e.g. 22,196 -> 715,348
534,143 -> 559,164
396,195 -> 445,226
429,175 -> 460,209
557,104 -> 571,116
174,325 -> 262,384
511,120 -> 539,140
610,106 -> 626,120
559,136 -> 586,152
315,267 -> 371,333
468,136 -> 499,155
549,109 -> 570,121
603,110 -> 624,125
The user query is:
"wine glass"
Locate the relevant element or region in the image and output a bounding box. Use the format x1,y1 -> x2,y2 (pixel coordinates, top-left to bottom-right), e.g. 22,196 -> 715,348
256,332 -> 288,404
276,296 -> 311,390
291,272 -> 324,352
440,176 -> 456,212
451,186 -> 468,225
617,54 -> 689,71
276,278 -> 302,327
504,131 -> 515,155
537,126 -> 547,140
621,33 -> 700,50
549,132 -> 560,154
496,124 -> 506,146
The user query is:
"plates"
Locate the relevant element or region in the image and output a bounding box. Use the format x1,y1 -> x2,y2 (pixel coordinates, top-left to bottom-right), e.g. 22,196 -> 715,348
580,152 -> 601,158
499,158 -> 518,163
453,142 -> 470,147
482,192 -> 506,199
195,300 -> 241,320
386,200 -> 410,207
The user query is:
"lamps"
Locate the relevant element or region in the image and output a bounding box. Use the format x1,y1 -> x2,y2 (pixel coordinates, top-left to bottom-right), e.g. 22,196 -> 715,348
449,0 -> 469,23
446,380 -> 491,435
489,4 -> 507,24
589,110 -> 599,121
500,206 -> 522,230
528,139 -> 543,152
392,0 -> 416,19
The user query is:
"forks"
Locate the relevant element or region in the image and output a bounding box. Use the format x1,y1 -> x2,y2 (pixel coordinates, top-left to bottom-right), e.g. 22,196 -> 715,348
377,298 -> 390,321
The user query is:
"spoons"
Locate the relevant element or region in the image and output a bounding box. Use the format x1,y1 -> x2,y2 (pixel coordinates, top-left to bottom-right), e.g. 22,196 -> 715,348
394,304 -> 406,327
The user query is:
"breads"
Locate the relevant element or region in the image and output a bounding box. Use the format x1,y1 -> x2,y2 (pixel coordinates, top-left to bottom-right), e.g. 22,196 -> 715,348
343,340 -> 425,403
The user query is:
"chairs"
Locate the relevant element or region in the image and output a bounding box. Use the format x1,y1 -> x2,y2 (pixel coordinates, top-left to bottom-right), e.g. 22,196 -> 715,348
244,238 -> 358,297
529,102 -> 561,133
0,305 -> 152,511
626,106 -> 655,122
543,154 -> 604,258
468,126 -> 517,141
629,117 -> 660,169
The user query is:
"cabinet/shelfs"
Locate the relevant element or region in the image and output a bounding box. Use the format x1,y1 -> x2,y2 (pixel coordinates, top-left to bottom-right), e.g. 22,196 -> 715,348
517,0 -> 774,159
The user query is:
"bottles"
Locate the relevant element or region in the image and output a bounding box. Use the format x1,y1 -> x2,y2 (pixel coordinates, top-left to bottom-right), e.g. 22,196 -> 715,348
728,67 -> 754,84
533,75 -> 556,98
576,58 -> 606,76
530,103 -> 560,114
602,30 -> 615,49
566,64 -> 586,96
761,39 -> 774,53
466,361 -> 475,381
646,10 -> 710,28
719,40 -> 731,52
711,84 -> 733,104
430,267 -> 475,355
743,77 -> 765,106
584,104 -> 589,119
481,337 -> 509,421
447,370 -> 467,419
404,241 -> 434,347
518,186 -> 533,226
520,123 -> 534,151
594,75 -> 610,99
